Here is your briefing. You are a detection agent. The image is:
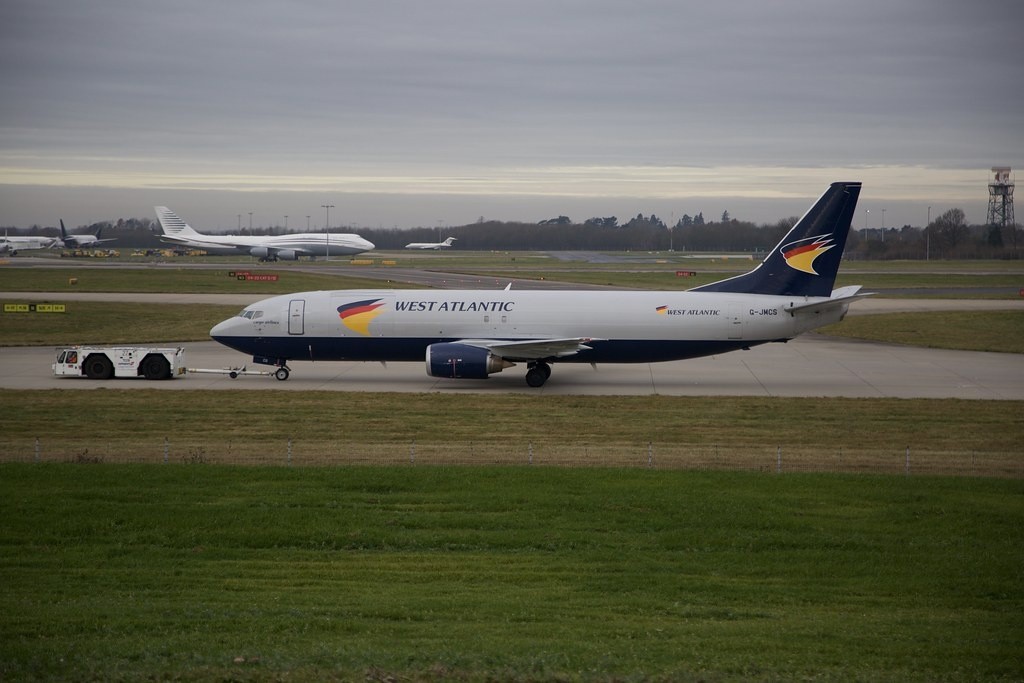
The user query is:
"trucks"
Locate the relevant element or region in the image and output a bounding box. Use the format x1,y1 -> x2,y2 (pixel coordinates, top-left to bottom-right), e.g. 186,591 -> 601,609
52,348 -> 184,381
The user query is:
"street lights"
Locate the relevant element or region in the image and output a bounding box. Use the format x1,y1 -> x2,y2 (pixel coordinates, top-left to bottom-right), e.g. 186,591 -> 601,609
882,207 -> 885,242
248,212 -> 254,235
284,215 -> 289,234
865,210 -> 870,242
669,210 -> 674,252
926,206 -> 931,261
305,216 -> 312,232
236,215 -> 242,235
321,204 -> 334,262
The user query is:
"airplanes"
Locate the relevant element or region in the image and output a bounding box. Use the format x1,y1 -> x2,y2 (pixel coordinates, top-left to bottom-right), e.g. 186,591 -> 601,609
405,237 -> 458,251
48,219 -> 119,250
0,228 -> 65,250
209,183 -> 886,388
0,230 -> 18,256
153,205 -> 375,262
